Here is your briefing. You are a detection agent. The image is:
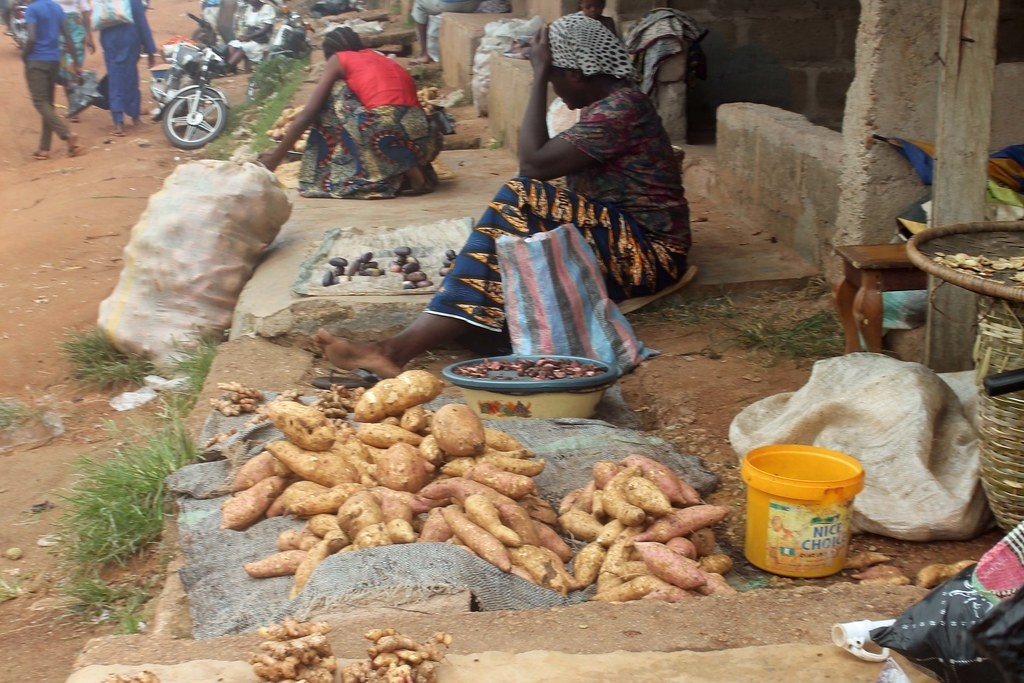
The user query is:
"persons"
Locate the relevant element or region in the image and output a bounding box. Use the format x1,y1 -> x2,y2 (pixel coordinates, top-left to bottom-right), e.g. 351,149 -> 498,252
579,0 -> 618,34
256,26 -> 441,198
311,13 -> 691,380
199,0 -> 276,75
22,0 -> 81,159
55,0 -> 95,121
100,0 -> 154,137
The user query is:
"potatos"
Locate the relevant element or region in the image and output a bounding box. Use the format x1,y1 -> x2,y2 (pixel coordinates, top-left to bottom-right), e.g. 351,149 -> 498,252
266,104 -> 312,154
222,369 -> 738,605
841,552 -> 977,588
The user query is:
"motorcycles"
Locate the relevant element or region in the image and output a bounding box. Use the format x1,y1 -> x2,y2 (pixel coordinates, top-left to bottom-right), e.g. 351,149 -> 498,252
3,0 -> 31,51
150,12 -> 238,151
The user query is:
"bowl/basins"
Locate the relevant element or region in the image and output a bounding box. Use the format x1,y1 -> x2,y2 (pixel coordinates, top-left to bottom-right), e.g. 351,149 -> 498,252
443,356 -> 618,419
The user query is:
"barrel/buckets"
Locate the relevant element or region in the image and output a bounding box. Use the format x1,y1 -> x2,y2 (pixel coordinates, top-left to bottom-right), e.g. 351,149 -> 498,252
742,444 -> 865,576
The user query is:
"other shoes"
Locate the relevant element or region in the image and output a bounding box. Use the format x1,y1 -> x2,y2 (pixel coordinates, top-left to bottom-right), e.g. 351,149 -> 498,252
70,116 -> 80,122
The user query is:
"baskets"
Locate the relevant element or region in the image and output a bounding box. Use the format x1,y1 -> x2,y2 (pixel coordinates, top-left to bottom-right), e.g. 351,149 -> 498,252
972,311 -> 1024,534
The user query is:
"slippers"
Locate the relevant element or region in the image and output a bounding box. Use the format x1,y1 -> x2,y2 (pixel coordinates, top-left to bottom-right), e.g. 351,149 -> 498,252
27,150 -> 48,160
409,56 -> 430,66
67,146 -> 86,157
312,368 -> 378,389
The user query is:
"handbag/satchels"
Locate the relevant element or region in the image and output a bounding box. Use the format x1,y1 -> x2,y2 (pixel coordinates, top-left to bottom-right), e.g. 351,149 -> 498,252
870,518 -> 1024,682
494,221 -> 661,384
88,0 -> 135,31
63,67 -> 104,119
